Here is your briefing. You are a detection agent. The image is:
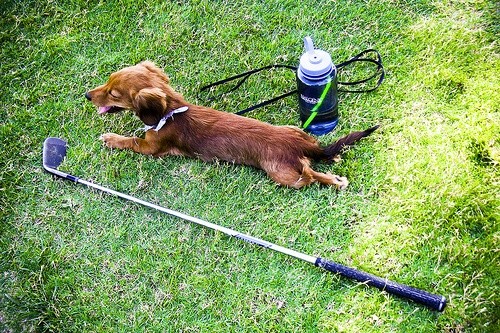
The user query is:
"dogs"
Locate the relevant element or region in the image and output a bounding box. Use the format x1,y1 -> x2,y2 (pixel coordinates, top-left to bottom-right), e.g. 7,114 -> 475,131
85,60 -> 381,191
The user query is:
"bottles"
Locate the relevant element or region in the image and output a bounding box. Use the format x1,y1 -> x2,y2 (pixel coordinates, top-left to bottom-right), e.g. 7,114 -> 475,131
296,36 -> 339,135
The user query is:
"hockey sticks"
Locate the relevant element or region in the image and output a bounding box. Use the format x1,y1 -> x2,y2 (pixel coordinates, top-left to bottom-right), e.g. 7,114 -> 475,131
42,136 -> 449,312
